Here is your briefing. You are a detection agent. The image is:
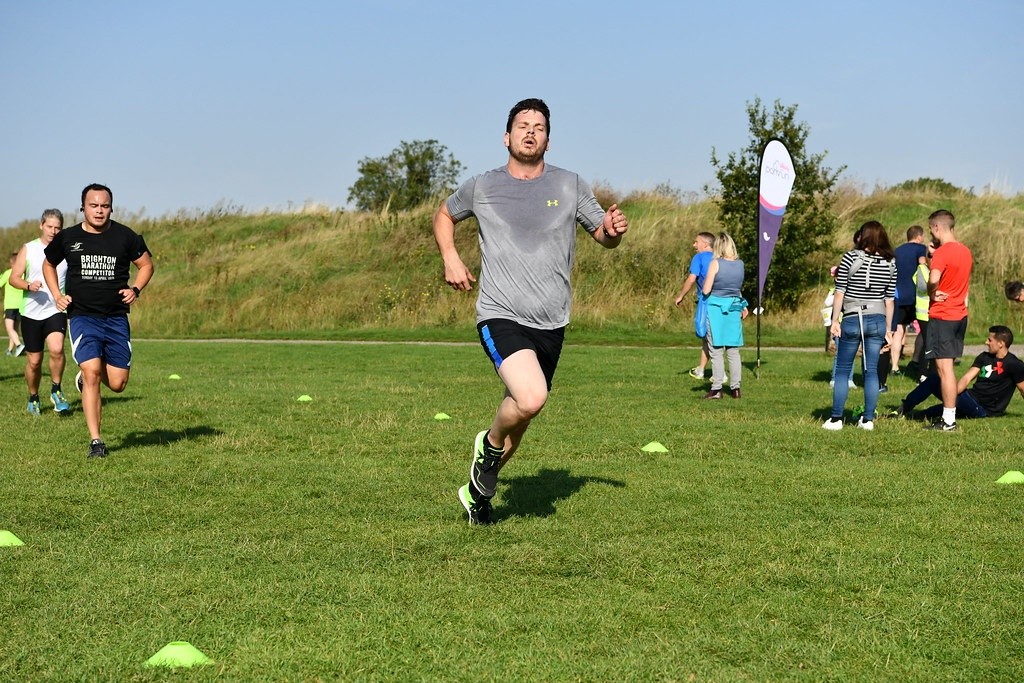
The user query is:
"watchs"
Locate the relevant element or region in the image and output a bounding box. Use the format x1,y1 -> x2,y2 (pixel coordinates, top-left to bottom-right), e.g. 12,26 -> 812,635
133,287 -> 140,297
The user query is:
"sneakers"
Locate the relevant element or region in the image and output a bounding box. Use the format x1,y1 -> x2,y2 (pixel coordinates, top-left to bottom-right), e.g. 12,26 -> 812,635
50,391 -> 70,412
854,415 -> 874,430
16,343 -> 25,358
28,400 -> 42,415
5,350 -> 13,355
471,429 -> 505,497
889,406 -> 912,419
708,373 -> 728,383
689,365 -> 704,379
458,481 -> 494,527
74,369 -> 85,392
87,438 -> 110,457
922,418 -> 957,433
822,417 -> 844,431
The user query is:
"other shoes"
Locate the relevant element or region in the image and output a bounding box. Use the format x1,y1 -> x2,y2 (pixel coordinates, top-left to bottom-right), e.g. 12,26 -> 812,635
909,362 -> 929,382
704,388 -> 725,399
730,388 -> 742,398
879,383 -> 888,392
889,369 -> 901,376
848,380 -> 857,390
829,380 -> 836,388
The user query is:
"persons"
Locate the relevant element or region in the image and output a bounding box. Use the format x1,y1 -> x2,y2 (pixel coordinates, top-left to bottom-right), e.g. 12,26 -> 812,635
922,210 -> 972,432
432,98 -> 628,527
0,253 -> 25,357
675,232 -> 748,383
43,183 -> 154,459
892,325 -> 1024,420
891,225 -> 923,375
821,221 -> 897,432
1004,281 -> 1024,302
9,208 -> 70,414
702,232 -> 748,400
912,242 -> 935,345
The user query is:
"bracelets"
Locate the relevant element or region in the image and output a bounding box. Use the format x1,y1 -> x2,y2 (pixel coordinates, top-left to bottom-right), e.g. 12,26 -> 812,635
28,283 -> 31,290
603,228 -> 609,238
